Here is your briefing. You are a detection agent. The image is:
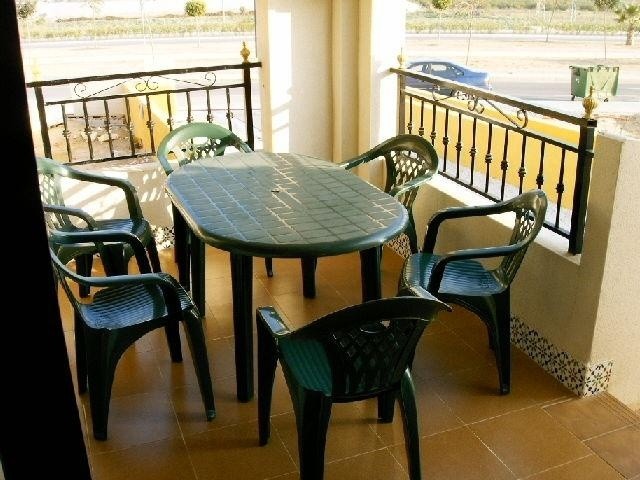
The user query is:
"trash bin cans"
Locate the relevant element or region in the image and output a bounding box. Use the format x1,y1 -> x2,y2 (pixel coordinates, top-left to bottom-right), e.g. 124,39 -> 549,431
569,64 -> 623,101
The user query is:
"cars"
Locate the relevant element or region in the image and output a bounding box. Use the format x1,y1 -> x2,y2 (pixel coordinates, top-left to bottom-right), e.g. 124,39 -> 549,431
401,61 -> 492,101
